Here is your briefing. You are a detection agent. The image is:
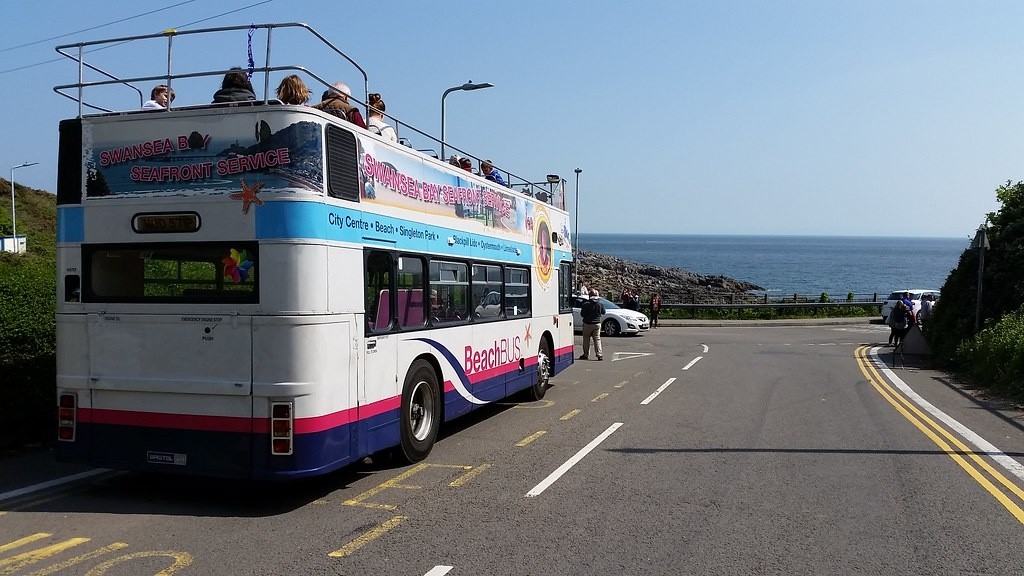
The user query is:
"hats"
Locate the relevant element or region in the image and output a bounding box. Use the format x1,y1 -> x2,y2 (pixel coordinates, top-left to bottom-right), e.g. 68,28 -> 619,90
450,155 -> 460,165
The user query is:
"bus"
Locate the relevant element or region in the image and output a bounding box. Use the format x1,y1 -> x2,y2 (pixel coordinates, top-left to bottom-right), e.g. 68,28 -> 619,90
53,23 -> 573,480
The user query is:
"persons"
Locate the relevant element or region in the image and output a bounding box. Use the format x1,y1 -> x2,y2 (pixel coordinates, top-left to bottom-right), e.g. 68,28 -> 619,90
650,294 -> 661,328
311,82 -> 365,127
481,160 -> 505,185
921,295 -> 933,326
364,93 -> 397,143
277,75 -> 310,105
888,292 -> 917,347
431,290 -> 437,305
142,85 -> 175,108
581,285 -> 599,296
622,291 -> 640,311
210,67 -> 256,102
450,155 -> 471,172
579,290 -> 606,361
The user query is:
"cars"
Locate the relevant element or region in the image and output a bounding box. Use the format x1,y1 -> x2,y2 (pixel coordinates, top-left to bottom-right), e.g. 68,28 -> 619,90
571,294 -> 649,337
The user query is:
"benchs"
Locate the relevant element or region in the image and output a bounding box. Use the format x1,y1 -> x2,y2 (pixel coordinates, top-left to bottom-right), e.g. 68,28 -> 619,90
77,98 -> 283,120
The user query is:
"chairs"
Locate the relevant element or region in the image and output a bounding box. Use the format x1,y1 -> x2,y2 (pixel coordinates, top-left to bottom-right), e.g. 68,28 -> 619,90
369,125 -> 382,136
398,138 -> 412,148
430,315 -> 461,324
417,149 -> 438,160
446,159 -> 496,183
323,109 -> 347,121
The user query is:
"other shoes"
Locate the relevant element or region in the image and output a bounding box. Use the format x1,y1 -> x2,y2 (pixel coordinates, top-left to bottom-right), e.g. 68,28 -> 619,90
598,357 -> 602,361
579,355 -> 589,360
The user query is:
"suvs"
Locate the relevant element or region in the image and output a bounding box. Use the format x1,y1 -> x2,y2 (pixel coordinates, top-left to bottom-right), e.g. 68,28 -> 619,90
882,289 -> 941,325
475,290 -> 528,319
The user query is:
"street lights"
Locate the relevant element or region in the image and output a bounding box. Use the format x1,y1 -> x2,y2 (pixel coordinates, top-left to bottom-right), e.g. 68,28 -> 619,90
441,80 -> 494,161
574,168 -> 582,293
10,162 -> 39,253
